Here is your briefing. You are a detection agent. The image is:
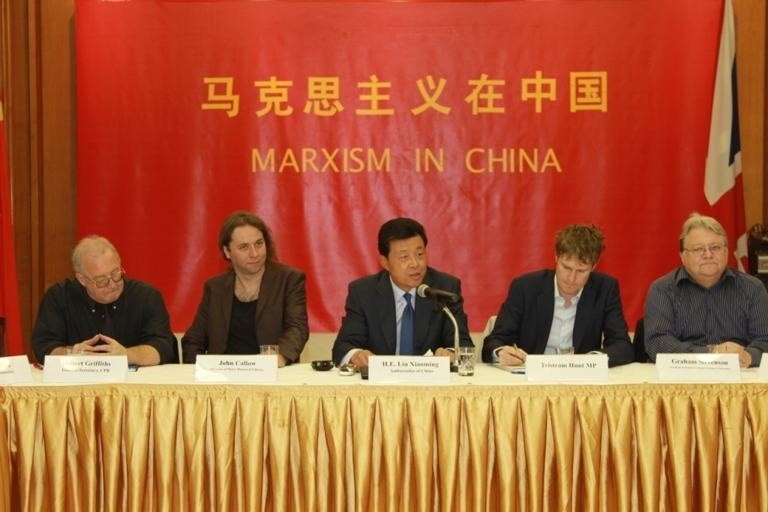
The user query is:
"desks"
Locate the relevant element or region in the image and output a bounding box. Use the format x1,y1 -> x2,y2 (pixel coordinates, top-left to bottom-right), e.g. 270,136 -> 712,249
1,363 -> 768,512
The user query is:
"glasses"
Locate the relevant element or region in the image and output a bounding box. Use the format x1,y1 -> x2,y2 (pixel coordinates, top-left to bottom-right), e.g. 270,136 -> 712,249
683,241 -> 728,254
84,266 -> 127,289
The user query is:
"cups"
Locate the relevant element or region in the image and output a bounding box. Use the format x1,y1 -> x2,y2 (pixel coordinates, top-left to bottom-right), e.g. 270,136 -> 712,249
455,346 -> 478,378
64,347 -> 88,357
554,345 -> 575,353
706,343 -> 728,353
259,344 -> 278,355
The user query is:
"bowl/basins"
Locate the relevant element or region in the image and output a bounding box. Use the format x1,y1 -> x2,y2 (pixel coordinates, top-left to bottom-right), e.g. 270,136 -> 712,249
311,360 -> 336,371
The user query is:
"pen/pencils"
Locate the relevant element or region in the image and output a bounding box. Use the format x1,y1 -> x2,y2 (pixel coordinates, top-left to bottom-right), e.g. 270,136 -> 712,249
513,343 -> 518,349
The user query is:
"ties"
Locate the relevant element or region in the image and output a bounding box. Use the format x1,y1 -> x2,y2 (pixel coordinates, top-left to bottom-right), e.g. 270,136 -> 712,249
399,293 -> 415,355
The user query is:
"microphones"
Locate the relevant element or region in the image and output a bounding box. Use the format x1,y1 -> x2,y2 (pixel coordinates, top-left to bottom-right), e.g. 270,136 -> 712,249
417,283 -> 460,304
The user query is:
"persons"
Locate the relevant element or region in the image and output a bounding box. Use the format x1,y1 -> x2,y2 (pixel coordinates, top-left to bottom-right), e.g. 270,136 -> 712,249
333,218 -> 476,372
181,210 -> 309,364
32,235 -> 179,366
643,213 -> 768,368
481,224 -> 636,367
